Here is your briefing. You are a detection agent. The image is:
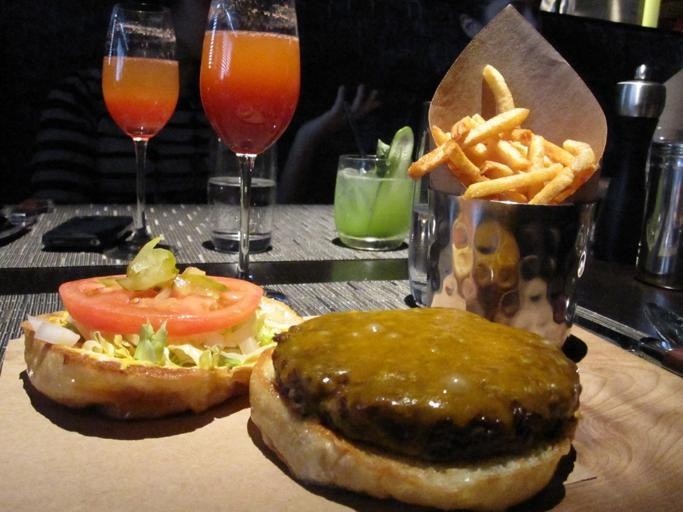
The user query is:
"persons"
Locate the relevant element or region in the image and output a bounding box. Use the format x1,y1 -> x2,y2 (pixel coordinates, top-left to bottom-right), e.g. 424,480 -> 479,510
29,4 -> 383,203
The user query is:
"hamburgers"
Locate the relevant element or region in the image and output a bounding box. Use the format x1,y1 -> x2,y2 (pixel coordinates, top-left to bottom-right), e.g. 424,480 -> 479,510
20,234 -> 301,421
249,308 -> 584,510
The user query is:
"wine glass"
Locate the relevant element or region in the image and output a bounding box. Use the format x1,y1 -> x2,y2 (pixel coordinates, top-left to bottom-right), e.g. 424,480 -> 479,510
101,0 -> 180,256
199,0 -> 301,311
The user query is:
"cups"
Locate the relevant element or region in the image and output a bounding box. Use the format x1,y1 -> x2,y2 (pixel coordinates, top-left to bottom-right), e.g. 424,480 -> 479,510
636,139 -> 683,292
206,134 -> 278,251
333,154 -> 420,253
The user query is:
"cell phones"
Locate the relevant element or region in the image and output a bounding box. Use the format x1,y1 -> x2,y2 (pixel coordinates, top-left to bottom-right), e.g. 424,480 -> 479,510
43,215 -> 132,245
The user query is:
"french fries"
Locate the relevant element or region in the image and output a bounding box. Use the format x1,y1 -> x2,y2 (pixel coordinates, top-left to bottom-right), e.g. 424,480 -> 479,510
407,64 -> 594,205
